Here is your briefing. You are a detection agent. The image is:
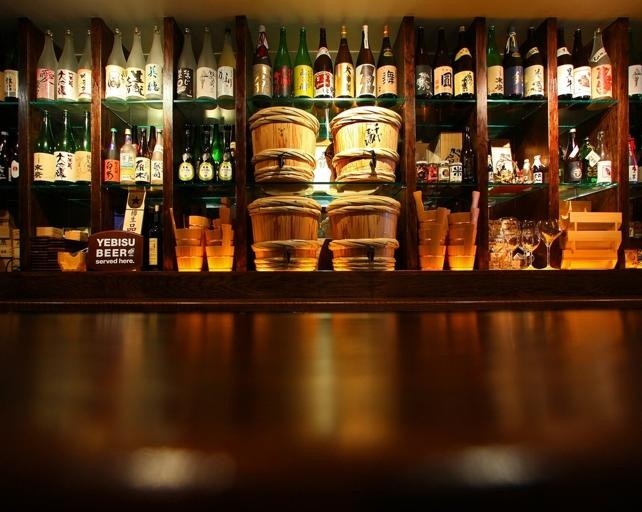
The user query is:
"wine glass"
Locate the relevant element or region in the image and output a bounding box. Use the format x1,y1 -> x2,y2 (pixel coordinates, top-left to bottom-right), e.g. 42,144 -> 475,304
487,217 -> 561,270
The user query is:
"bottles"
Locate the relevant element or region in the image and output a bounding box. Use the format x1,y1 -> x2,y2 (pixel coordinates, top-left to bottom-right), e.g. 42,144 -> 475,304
245,21 -> 403,110
0,21 -> 28,104
626,25 -> 641,101
144,205 -> 162,270
484,24 -> 548,101
104,24 -> 165,111
627,134 -> 640,183
30,108 -> 91,189
554,21 -> 615,101
487,152 -> 545,191
414,21 -> 479,101
1,105 -> 24,185
414,125 -> 476,189
36,26 -> 94,114
170,22 -> 237,121
560,127 -> 614,189
103,126 -> 163,195
177,122 -> 238,193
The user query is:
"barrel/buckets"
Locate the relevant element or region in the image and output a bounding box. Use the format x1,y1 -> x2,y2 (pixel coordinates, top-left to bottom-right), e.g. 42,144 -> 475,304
327,196 -> 401,271
249,197 -> 323,272
322,142 -> 383,194
329,105 -> 402,182
250,106 -> 319,182
261,181 -> 316,197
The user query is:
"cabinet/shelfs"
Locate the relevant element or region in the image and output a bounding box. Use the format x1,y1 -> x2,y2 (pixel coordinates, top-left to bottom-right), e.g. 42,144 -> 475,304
18,14 -> 631,270
